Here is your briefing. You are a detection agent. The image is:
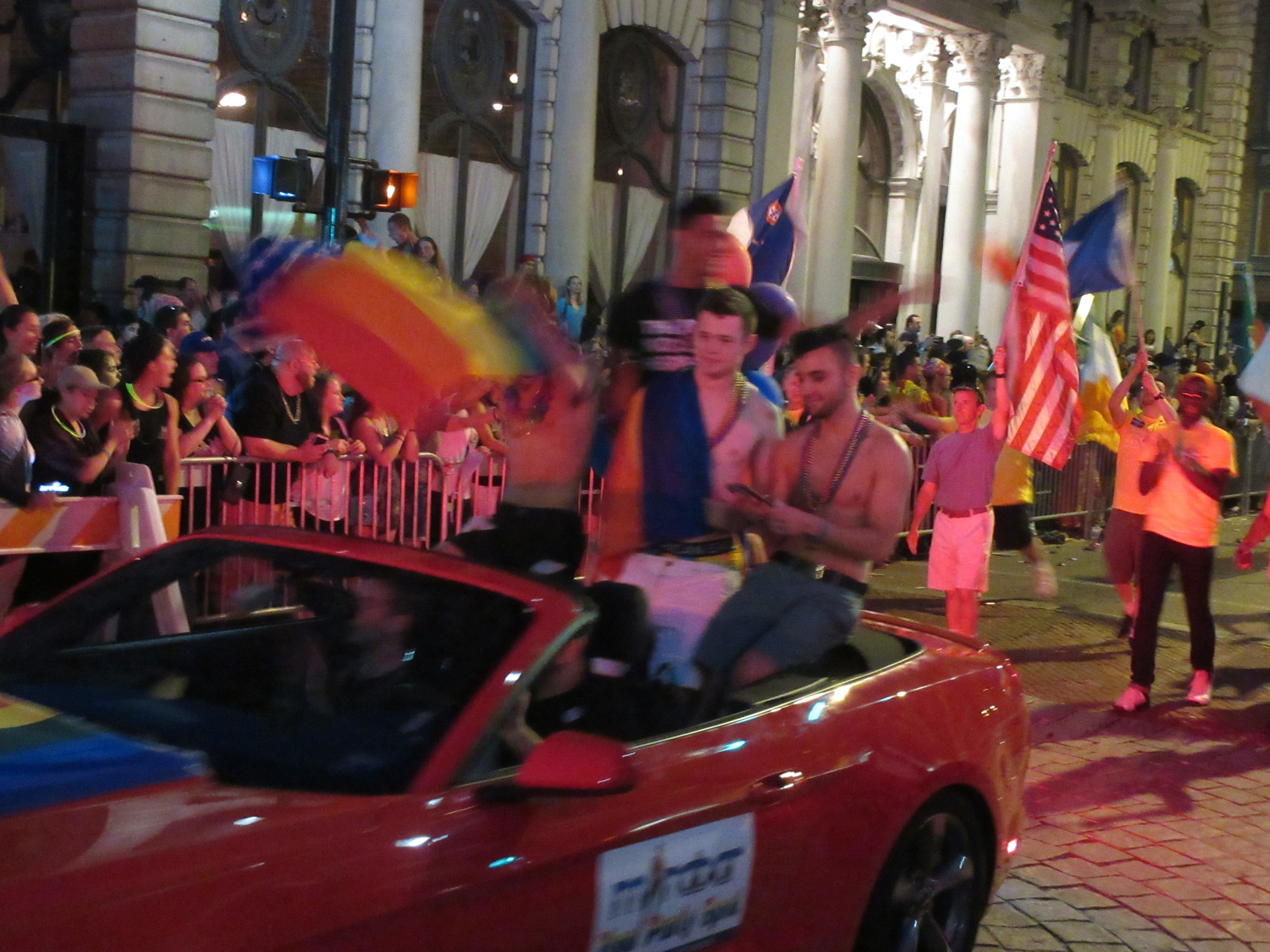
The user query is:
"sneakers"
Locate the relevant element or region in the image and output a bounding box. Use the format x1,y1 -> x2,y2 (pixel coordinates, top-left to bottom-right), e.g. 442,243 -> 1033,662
1113,686 -> 1151,712
1187,669 -> 1214,704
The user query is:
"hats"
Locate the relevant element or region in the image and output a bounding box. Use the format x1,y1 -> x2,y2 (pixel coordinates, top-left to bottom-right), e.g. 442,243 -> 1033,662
1175,372 -> 1218,399
788,323 -> 852,361
922,357 -> 947,378
180,331 -> 222,353
57,365 -> 111,392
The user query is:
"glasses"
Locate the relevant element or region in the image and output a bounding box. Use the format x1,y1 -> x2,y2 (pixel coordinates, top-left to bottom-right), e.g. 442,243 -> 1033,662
24,373 -> 40,384
190,376 -> 210,385
943,373 -> 952,377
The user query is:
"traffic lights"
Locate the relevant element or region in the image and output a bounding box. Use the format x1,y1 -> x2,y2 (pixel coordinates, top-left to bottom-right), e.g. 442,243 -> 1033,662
252,157 -> 304,202
374,171 -> 407,211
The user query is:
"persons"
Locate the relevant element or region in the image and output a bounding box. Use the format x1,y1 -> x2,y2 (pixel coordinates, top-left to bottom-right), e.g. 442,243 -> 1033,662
0,167 -> 1270,806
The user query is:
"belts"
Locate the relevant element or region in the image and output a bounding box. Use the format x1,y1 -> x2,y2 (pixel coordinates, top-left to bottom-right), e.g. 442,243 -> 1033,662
653,536 -> 735,559
770,549 -> 868,596
937,504 -> 993,518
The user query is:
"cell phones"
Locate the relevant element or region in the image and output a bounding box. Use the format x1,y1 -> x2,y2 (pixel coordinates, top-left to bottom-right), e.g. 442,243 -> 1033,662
725,483 -> 773,507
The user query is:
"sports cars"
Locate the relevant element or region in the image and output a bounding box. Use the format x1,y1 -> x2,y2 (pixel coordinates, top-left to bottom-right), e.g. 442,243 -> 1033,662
0,522 -> 1033,952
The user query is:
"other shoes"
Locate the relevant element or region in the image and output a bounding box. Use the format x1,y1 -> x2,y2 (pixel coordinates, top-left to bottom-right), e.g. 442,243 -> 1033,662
1118,613 -> 1133,637
1035,562 -> 1057,597
